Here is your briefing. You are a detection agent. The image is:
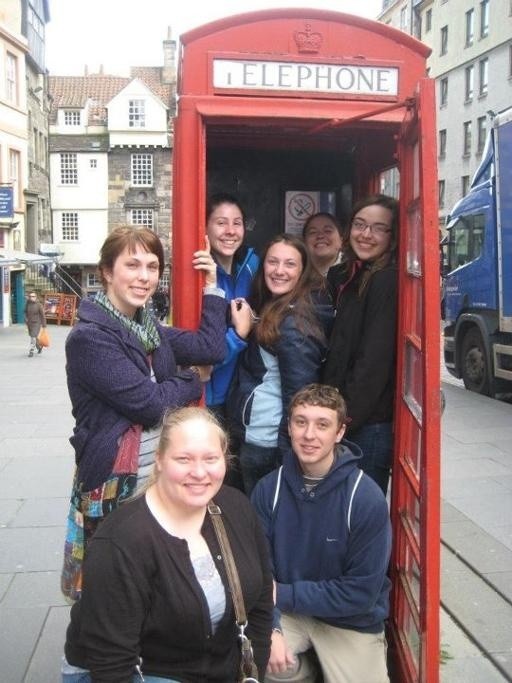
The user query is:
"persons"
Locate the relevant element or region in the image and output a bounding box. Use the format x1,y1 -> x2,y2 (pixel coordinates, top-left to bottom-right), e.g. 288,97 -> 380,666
61,405 -> 273,683
301,212 -> 352,335
204,193 -> 262,407
62,226 -> 227,608
236,234 -> 329,498
321,195 -> 398,498
249,385 -> 392,681
24,292 -> 47,357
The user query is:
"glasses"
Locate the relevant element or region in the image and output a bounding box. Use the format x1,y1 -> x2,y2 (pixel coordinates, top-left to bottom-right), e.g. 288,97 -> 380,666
348,219 -> 393,236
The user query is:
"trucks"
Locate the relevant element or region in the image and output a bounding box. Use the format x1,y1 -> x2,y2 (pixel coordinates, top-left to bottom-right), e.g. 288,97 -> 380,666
438,105 -> 512,399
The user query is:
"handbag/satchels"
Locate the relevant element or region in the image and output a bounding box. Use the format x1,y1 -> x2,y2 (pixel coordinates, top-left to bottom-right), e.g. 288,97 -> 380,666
36,329 -> 49,348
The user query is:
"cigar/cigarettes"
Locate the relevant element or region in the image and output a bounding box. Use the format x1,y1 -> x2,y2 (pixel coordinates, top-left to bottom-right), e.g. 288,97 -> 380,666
298,202 -> 312,216
